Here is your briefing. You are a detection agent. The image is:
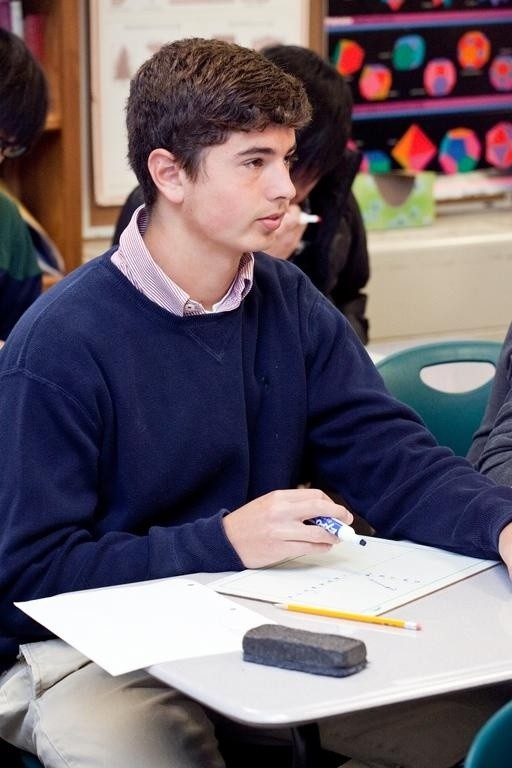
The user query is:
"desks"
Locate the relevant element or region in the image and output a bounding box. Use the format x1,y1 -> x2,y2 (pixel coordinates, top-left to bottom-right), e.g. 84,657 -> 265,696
125,536 -> 511,767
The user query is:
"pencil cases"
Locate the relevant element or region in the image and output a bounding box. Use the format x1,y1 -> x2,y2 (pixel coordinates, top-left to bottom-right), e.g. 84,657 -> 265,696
241,624 -> 367,677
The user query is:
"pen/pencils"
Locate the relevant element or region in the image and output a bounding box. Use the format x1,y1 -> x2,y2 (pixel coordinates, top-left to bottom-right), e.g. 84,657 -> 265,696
297,211 -> 326,226
274,602 -> 424,631
306,515 -> 366,546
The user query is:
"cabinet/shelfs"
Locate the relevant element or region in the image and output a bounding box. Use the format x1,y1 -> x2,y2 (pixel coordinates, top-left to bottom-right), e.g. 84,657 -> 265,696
305,0 -> 512,220
1,0 -> 82,288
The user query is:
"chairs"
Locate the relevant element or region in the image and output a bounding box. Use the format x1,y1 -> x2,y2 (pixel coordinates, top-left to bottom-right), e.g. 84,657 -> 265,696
461,699 -> 511,767
365,341 -> 505,455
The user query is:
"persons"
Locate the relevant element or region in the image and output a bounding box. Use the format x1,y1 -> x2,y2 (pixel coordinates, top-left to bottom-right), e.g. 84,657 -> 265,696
463,324 -> 511,488
111,46 -> 371,344
1,28 -> 64,348
3,36 -> 511,768
